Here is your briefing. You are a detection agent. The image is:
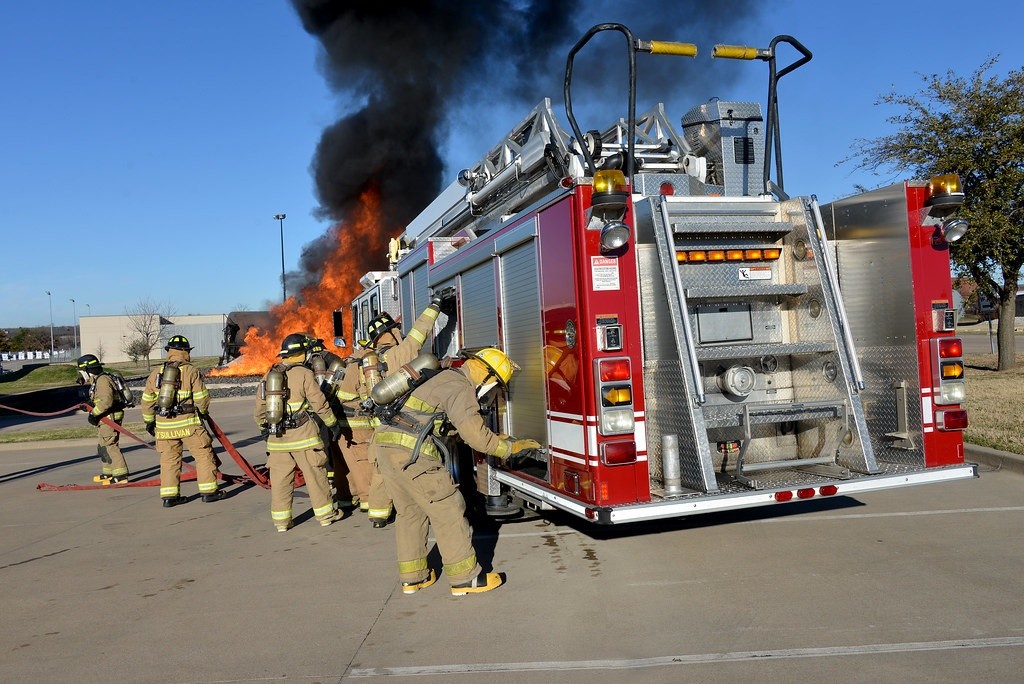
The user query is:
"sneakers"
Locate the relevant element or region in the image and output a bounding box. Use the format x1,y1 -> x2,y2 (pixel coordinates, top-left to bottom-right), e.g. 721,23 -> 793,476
271,472 -> 395,532
402,568 -> 436,594
450,572 -> 502,596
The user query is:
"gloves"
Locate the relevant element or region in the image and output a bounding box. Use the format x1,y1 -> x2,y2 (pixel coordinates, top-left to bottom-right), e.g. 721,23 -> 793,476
330,422 -> 341,441
431,290 -> 442,308
146,422 -> 156,437
201,413 -> 208,420
80,401 -> 93,412
511,439 -> 541,454
499,434 -> 518,442
88,414 -> 98,426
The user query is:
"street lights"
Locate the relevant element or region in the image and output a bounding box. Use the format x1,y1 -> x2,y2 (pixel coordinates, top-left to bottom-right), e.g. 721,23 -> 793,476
273,214 -> 286,302
47,291 -> 53,355
70,299 -> 77,349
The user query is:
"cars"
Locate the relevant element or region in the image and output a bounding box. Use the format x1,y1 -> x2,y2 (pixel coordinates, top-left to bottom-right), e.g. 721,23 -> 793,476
3,369 -> 13,374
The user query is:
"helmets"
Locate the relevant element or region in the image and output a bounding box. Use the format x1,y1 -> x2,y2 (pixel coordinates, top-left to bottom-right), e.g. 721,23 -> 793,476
364,311 -> 400,349
76,354 -> 104,370
276,334 -> 313,357
165,335 -> 195,352
358,340 -> 373,349
461,347 -> 513,392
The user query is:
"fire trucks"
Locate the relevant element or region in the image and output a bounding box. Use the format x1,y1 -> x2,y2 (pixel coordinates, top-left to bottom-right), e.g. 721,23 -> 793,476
334,22 -> 981,526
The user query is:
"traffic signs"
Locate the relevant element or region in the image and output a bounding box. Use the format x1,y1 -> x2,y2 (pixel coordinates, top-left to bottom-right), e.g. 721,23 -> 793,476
978,288 -> 997,313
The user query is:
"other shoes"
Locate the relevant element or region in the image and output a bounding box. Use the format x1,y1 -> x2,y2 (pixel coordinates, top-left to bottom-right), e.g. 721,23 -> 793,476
103,475 -> 129,485
202,490 -> 226,502
163,496 -> 188,507
93,474 -> 113,482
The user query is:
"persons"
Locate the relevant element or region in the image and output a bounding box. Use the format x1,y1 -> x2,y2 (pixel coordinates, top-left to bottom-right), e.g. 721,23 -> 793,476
142,335 -> 228,506
255,290 -> 444,533
77,354 -> 129,485
371,349 -> 540,596
